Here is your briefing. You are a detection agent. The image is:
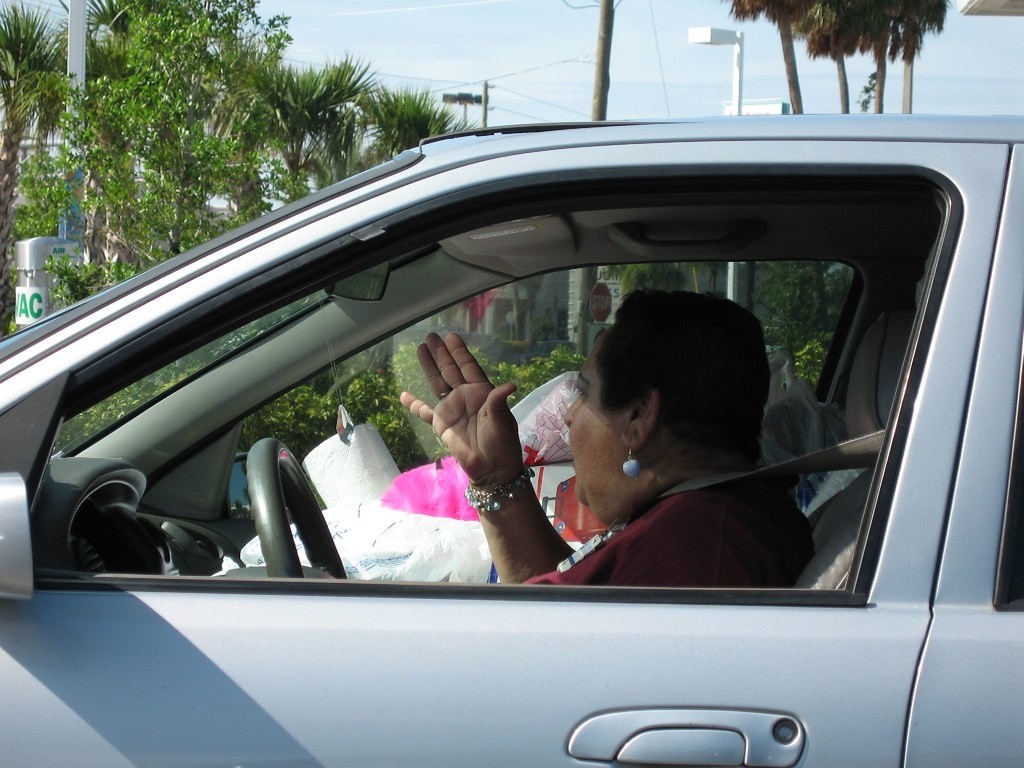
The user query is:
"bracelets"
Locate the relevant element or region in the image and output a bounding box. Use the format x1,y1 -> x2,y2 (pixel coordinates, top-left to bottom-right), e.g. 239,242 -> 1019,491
464,465 -> 535,510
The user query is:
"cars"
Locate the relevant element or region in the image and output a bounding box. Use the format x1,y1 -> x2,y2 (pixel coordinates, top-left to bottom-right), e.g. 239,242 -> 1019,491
1,113 -> 1024,767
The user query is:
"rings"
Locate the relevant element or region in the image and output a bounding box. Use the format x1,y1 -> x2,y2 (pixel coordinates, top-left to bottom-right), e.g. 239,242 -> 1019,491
437,388 -> 453,400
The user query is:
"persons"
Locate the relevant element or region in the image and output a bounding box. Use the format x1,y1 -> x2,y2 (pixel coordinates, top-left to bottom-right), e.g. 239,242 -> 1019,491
399,290 -> 816,587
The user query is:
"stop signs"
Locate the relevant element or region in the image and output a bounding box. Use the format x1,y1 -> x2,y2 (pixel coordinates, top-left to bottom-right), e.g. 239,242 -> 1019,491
591,281 -> 611,324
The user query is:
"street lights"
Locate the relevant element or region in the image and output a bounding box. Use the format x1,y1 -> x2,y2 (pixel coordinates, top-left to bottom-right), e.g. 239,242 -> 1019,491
689,26 -> 747,116
442,92 -> 488,127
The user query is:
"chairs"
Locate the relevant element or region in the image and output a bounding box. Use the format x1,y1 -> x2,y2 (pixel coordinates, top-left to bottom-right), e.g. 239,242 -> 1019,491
789,307 -> 916,591
